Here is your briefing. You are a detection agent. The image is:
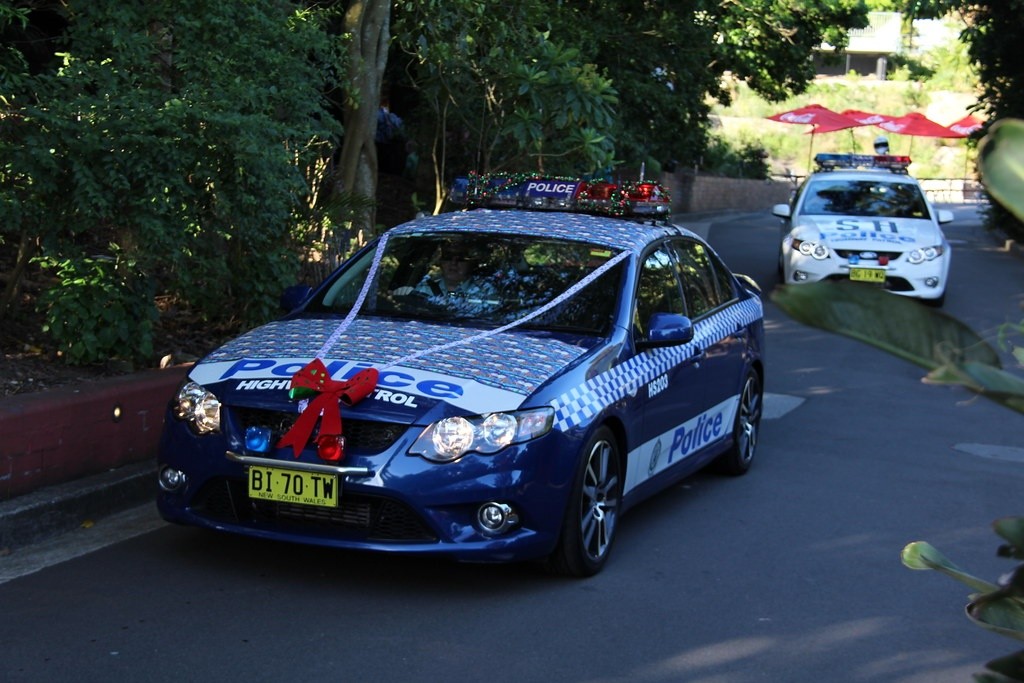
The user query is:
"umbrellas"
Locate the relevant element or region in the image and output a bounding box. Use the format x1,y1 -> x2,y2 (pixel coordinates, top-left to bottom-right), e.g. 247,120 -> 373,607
877,112 -> 965,160
947,115 -> 988,138
767,104 -> 860,173
839,110 -> 892,153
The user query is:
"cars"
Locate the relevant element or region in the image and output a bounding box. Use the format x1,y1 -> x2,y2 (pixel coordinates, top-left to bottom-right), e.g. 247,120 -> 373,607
771,152 -> 953,308
154,175 -> 765,574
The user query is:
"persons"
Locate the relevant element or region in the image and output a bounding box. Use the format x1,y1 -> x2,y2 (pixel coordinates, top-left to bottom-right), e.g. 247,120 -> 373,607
412,239 -> 503,318
